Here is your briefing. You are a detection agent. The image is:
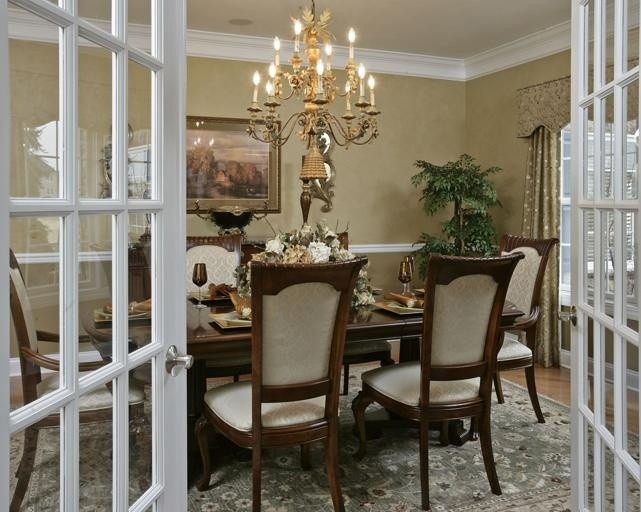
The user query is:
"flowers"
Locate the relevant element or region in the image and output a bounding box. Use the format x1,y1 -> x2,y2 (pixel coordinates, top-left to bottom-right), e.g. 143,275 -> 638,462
235,227 -> 377,308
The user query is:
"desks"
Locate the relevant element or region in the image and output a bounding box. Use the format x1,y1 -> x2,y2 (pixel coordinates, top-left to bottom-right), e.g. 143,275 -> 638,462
71,294 -> 527,446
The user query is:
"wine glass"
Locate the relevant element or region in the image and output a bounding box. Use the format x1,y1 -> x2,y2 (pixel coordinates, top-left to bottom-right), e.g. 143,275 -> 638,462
192,263 -> 207,309
398,256 -> 415,296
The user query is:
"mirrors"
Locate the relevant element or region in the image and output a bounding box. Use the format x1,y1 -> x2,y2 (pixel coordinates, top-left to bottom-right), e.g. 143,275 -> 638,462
310,128 -> 337,213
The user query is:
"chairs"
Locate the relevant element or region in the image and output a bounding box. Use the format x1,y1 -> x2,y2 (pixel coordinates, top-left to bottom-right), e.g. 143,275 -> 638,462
137,232 -> 245,386
335,231 -> 392,398
5,245 -> 150,511
195,256 -> 371,511
350,248 -> 528,510
491,237 -> 559,425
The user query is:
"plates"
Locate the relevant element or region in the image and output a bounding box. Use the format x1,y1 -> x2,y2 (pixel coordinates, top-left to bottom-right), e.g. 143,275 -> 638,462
208,311 -> 254,330
94,306 -> 149,319
192,291 -> 232,300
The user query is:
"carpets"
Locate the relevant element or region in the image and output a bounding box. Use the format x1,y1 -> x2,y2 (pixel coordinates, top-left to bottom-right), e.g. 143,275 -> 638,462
8,367 -> 640,512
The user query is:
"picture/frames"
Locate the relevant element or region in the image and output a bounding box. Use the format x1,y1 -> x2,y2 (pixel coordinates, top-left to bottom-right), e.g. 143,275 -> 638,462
186,116 -> 281,214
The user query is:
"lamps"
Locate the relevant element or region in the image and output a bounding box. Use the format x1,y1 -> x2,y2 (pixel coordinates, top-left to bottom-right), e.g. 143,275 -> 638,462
247,0 -> 381,180
